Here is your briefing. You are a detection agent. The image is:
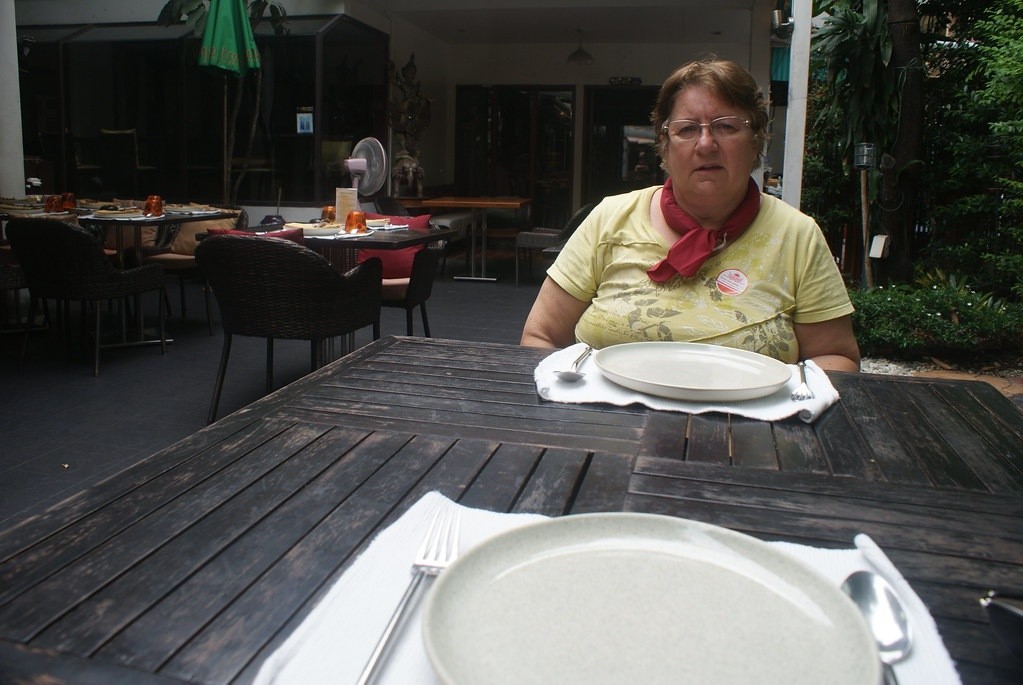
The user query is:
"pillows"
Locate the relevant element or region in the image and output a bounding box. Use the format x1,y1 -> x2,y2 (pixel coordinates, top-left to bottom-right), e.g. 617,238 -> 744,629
169,202 -> 242,254
358,210 -> 433,279
104,199 -> 166,249
207,227 -> 305,246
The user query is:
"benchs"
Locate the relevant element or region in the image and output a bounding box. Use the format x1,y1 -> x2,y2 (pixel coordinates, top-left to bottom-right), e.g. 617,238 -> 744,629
405,185 -> 539,254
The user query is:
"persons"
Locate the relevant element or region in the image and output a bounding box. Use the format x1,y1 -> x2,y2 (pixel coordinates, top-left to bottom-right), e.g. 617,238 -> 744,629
763,171 -> 772,193
520,51 -> 859,372
392,51 -> 421,158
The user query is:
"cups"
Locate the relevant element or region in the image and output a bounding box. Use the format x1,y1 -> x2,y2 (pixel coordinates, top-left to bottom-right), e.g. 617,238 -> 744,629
346,211 -> 366,234
143,196 -> 163,217
45,196 -> 63,213
321,206 -> 336,222
61,193 -> 76,208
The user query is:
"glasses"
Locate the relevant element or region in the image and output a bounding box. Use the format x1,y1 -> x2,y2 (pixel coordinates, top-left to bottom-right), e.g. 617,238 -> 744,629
662,116 -> 754,142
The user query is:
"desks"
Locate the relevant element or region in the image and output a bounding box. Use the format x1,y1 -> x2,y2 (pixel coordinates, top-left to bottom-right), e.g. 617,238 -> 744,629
195,222 -> 458,371
389,192 -> 535,282
0,336 -> 1023,685
0,207 -> 238,349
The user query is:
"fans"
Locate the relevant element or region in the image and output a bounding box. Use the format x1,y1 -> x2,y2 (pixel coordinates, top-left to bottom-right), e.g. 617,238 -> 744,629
344,137 -> 389,197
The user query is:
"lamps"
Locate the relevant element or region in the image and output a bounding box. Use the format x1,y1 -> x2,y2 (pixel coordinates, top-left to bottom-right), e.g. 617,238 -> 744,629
568,33 -> 593,63
771,10 -> 794,30
853,143 -> 878,170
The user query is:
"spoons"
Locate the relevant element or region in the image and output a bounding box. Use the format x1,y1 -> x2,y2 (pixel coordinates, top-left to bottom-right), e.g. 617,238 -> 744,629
842,571 -> 913,685
557,347 -> 591,380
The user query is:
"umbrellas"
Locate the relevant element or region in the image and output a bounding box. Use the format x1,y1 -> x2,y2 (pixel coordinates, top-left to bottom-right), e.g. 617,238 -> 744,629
198,0 -> 261,203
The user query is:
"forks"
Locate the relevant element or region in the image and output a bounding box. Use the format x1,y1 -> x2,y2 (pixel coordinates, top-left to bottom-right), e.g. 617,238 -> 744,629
791,361 -> 814,401
356,511 -> 459,685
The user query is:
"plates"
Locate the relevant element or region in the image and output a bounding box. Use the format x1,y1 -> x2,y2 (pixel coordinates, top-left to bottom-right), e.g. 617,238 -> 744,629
593,341 -> 793,401
422,511 -> 879,685
367,217 -> 391,226
282,224 -> 341,234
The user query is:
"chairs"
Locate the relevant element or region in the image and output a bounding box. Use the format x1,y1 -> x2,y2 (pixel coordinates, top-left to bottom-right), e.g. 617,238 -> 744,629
466,172 -> 593,289
0,204 -> 458,424
